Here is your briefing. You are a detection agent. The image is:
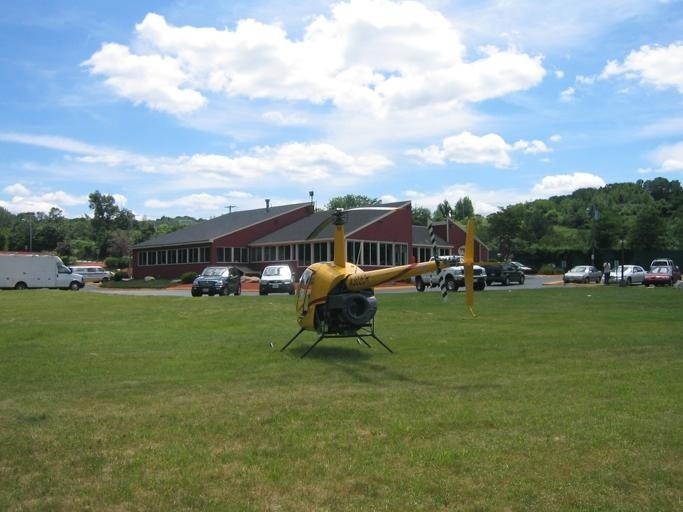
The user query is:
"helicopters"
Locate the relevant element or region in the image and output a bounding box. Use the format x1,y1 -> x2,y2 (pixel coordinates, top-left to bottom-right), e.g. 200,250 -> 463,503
280,203 -> 476,357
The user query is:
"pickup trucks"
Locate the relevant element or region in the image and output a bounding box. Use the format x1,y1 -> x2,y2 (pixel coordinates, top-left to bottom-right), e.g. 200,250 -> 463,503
411,255 -> 487,291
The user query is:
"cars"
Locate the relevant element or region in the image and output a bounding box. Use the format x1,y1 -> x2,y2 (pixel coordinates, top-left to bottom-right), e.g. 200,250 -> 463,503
259,264 -> 295,296
191,265 -> 241,296
485,261 -> 532,285
563,257 -> 681,287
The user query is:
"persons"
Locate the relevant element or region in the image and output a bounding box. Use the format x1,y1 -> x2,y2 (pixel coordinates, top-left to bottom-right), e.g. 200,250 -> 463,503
603,260 -> 610,284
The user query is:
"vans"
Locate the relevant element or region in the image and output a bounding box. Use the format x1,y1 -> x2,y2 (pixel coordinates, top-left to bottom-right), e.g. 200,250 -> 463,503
0,254 -> 85,291
68,265 -> 115,283
